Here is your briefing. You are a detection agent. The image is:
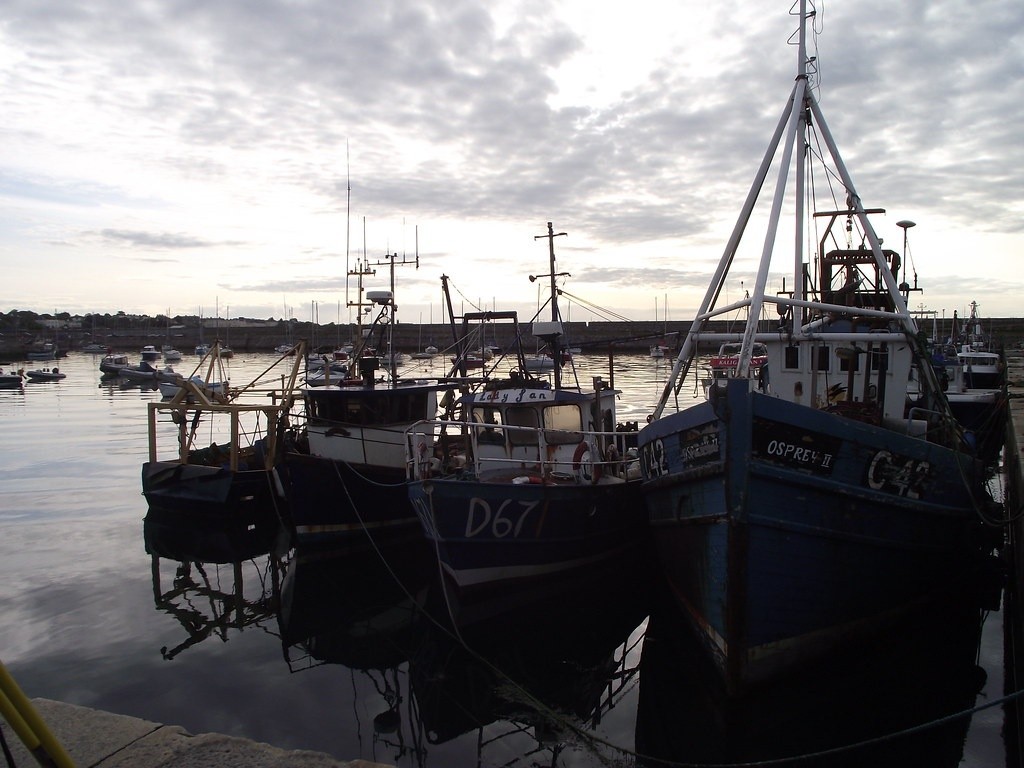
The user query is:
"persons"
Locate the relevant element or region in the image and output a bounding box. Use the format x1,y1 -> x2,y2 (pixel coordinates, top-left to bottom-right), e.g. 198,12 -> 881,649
479,420 -> 504,442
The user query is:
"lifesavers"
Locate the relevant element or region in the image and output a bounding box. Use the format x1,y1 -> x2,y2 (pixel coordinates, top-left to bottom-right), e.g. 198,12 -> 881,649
606,444 -> 620,478
511,475 -> 558,486
572,440 -> 603,487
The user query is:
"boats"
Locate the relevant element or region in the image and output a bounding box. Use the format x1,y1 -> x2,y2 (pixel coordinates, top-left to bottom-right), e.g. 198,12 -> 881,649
164,349 -> 184,360
82,344 -> 110,353
0,374 -> 22,388
26,368 -> 66,381
140,344 -> 161,360
276,253 -> 473,550
637,0 -> 993,698
99,354 -> 134,373
27,343 -> 56,360
195,343 -> 212,355
405,221 -> 648,589
906,301 -> 1002,425
141,336 -> 330,527
157,376 -> 229,399
119,361 -> 183,382
710,342 -> 768,378
275,344 -> 572,387
219,345 -> 233,358
